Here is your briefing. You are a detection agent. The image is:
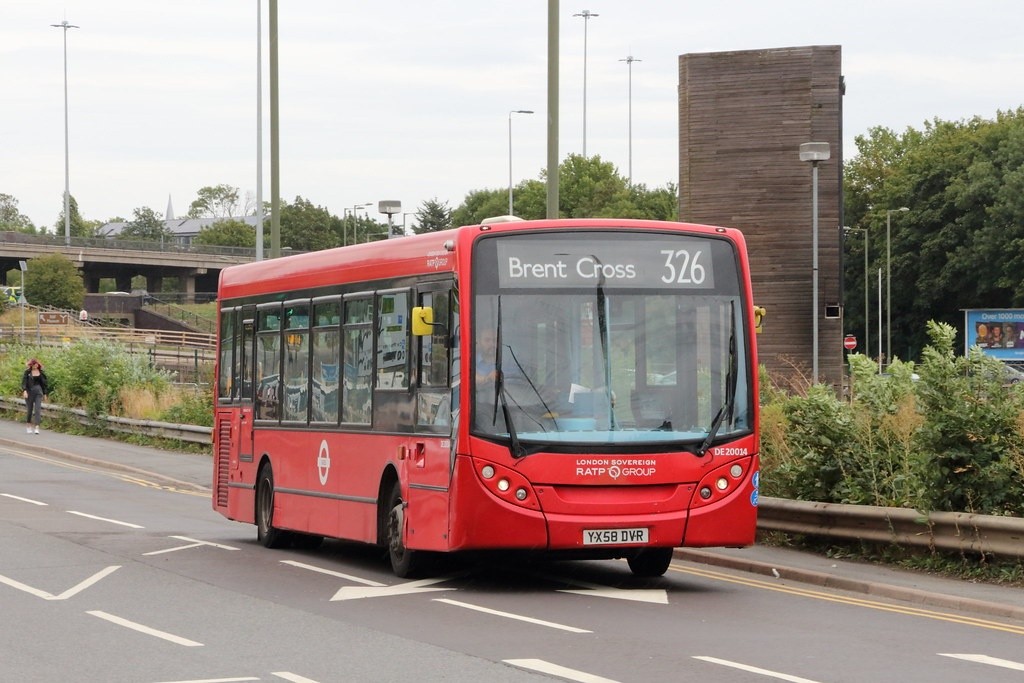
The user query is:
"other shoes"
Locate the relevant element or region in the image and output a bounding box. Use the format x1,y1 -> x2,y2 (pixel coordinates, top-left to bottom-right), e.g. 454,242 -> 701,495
26,424 -> 32,434
34,425 -> 40,434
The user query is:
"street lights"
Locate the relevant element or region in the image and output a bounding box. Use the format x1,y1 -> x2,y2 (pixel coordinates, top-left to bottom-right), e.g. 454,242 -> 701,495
509,110 -> 534,216
50,20 -> 80,245
354,203 -> 373,245
888,207 -> 909,367
800,141 -> 830,388
573,9 -> 599,160
379,201 -> 401,240
344,207 -> 365,247
843,226 -> 869,355
619,56 -> 642,189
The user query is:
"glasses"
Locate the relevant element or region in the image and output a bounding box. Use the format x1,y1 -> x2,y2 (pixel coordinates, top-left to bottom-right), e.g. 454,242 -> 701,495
480,335 -> 497,342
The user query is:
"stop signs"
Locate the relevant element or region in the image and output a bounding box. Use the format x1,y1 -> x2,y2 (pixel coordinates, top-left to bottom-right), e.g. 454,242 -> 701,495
844,337 -> 857,349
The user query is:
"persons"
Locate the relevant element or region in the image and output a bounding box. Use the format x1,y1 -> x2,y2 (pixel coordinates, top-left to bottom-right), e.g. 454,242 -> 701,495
448,328 -> 616,432
989,324 -> 1015,349
21,359 -> 51,435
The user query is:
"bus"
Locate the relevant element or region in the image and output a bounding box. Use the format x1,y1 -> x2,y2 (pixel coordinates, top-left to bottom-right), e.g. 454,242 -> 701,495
212,217 -> 766,577
